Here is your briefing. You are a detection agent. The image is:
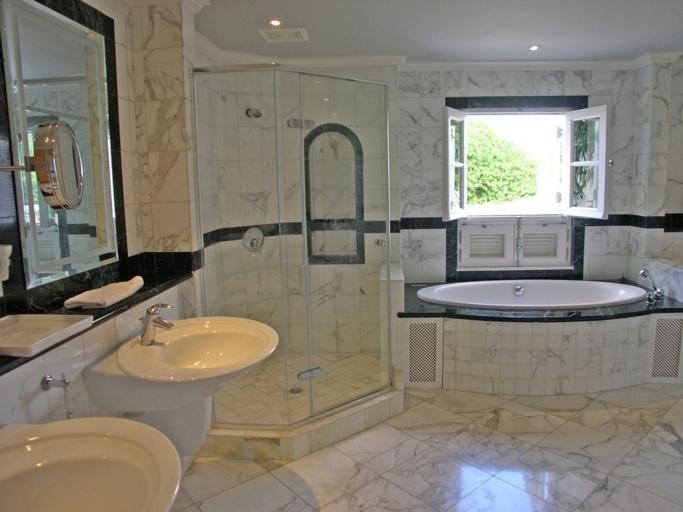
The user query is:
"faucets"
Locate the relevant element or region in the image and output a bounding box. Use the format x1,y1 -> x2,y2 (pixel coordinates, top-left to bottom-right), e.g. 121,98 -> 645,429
639,269 -> 665,302
139,302 -> 174,347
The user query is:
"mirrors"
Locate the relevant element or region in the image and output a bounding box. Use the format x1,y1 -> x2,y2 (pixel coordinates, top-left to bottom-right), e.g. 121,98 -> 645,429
0,0 -> 119,289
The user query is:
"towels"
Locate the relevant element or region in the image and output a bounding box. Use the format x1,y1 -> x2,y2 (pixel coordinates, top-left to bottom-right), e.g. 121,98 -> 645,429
64,276 -> 144,310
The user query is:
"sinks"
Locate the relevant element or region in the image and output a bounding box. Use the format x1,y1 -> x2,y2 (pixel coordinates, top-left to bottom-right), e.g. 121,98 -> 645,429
0,418 -> 181,511
83,310 -> 281,413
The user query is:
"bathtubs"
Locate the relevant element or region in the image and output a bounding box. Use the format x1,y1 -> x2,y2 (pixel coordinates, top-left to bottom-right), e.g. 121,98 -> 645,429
416,278 -> 648,310
